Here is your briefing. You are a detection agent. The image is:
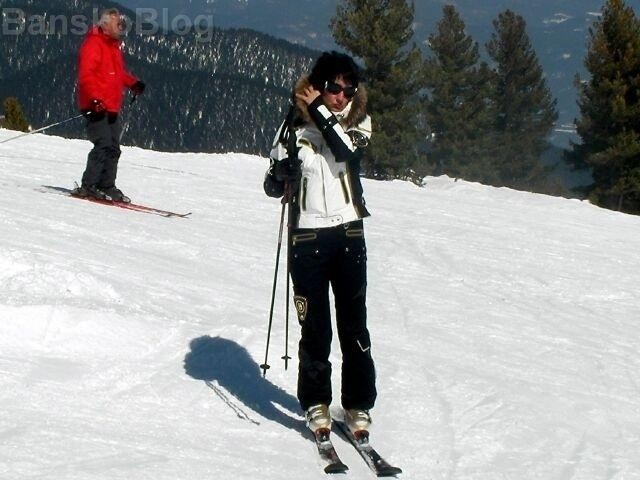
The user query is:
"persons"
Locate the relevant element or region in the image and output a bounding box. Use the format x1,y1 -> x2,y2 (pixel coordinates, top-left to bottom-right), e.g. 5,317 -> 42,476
76,8 -> 145,205
262,50 -> 379,445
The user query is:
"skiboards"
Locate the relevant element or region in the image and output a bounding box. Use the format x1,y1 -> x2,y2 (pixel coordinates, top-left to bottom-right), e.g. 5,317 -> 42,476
305,406 -> 403,476
32,181 -> 191,219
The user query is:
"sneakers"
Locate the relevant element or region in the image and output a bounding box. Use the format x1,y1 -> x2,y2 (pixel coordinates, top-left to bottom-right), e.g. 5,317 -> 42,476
306,404 -> 332,434
101,186 -> 131,204
345,409 -> 372,432
80,185 -> 112,201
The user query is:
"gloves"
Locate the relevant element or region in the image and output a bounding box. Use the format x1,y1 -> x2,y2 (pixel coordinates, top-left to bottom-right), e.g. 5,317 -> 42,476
89,101 -> 105,113
275,157 -> 302,181
130,82 -> 146,95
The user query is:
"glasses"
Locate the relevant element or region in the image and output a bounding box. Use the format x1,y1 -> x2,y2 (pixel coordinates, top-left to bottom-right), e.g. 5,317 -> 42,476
325,82 -> 358,98
